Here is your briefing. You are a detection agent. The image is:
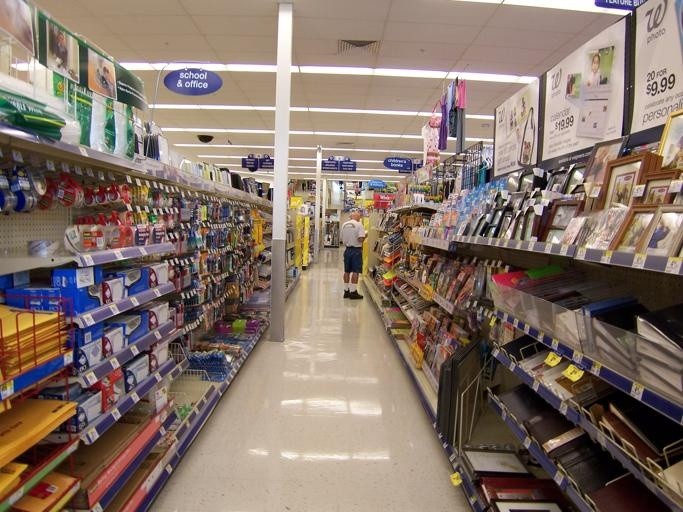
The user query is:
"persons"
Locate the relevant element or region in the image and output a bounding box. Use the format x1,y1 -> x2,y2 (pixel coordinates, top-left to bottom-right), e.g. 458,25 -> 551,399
54,32 -> 67,69
339,211 -> 368,299
589,55 -> 603,85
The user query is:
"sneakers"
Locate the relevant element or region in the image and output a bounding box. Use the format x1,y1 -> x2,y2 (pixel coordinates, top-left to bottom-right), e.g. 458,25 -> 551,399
350,290 -> 363,299
344,288 -> 350,298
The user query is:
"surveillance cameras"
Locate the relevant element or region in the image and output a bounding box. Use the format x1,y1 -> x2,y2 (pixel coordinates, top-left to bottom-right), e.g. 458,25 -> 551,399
197,135 -> 215,144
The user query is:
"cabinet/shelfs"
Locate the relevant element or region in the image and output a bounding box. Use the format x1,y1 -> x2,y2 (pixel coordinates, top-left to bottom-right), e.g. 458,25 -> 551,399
324,208 -> 340,248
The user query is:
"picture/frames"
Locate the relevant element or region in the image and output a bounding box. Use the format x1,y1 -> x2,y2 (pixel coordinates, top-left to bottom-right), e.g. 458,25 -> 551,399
658,110 -> 683,170
